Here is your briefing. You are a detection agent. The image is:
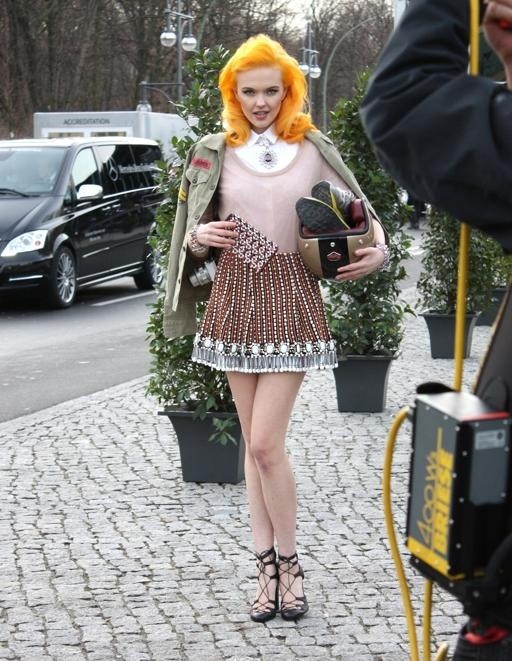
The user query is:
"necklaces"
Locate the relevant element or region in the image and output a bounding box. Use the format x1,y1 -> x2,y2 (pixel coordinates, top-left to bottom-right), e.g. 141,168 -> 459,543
257,140 -> 277,169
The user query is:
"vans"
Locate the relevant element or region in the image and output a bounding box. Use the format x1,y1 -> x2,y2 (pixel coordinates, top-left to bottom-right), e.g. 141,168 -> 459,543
0,133 -> 169,303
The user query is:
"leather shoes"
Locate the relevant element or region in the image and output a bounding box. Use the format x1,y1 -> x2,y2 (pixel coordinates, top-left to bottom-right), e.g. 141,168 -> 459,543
217,212 -> 277,274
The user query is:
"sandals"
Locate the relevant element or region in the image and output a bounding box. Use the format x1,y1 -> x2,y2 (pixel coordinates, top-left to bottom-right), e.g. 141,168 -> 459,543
251,546 -> 279,623
279,553 -> 309,620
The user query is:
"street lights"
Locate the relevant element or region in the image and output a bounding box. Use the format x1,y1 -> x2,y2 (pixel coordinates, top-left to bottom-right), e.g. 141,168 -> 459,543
323,12 -> 383,136
134,5 -> 199,122
297,22 -> 322,130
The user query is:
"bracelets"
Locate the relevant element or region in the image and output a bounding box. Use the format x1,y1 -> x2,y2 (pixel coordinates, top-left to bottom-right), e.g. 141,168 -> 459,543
377,243 -> 390,269
188,220 -> 205,254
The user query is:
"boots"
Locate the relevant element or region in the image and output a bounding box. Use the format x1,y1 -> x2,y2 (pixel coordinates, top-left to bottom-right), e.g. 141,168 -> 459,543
295,180 -> 356,235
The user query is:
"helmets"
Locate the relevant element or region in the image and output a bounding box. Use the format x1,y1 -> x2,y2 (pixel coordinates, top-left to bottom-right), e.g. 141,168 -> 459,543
298,198 -> 385,282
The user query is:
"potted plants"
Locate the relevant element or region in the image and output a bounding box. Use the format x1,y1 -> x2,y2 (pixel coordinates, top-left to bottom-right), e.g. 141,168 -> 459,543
318,71 -> 418,413
415,207 -> 512,360
142,43 -> 246,484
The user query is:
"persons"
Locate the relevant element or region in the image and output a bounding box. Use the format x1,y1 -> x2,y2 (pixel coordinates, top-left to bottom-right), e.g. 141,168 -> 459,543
357,0 -> 512,661
160,30 -> 391,623
